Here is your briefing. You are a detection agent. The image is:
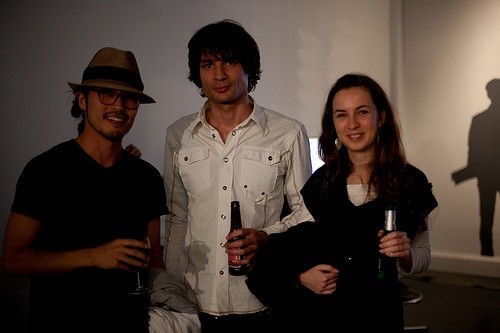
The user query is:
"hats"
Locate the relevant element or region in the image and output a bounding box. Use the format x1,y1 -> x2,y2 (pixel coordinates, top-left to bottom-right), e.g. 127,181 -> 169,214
66,46 -> 157,113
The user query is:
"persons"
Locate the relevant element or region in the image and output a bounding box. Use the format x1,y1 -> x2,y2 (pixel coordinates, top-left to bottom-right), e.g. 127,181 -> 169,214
0,47 -> 168,333
245,73 -> 437,333
163,19 -> 314,333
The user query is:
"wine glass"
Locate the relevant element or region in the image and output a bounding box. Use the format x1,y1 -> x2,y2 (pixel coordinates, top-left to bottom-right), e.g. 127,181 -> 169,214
122,236 -> 153,296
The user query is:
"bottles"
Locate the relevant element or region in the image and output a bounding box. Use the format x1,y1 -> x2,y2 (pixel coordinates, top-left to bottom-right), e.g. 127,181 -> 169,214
377,204 -> 403,298
227,201 -> 248,276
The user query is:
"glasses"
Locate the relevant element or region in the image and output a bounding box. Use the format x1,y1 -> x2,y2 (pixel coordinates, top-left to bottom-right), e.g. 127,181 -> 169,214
88,87 -> 141,110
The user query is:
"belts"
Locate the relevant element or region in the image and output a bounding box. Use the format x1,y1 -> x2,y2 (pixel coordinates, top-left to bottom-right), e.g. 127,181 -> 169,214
204,312 -> 265,320
